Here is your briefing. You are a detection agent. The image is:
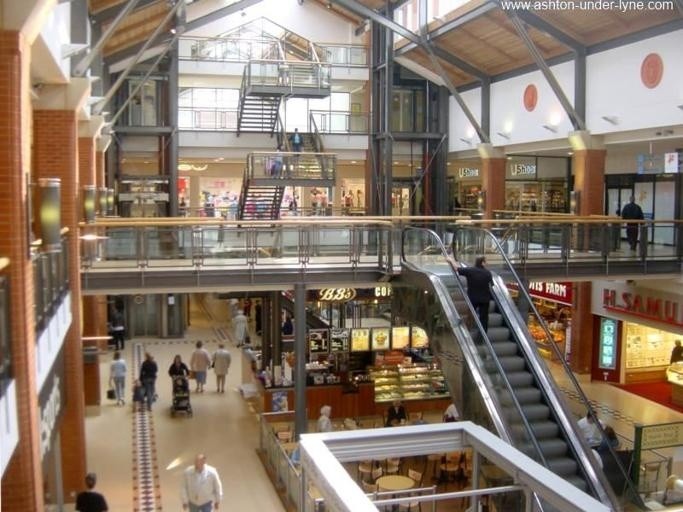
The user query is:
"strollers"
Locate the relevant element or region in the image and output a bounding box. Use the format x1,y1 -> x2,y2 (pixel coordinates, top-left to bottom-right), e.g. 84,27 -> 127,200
170,376 -> 194,418
132,377 -> 155,411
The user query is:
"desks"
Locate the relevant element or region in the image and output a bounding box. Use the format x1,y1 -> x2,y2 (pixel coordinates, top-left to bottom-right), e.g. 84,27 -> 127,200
391,420 -> 429,427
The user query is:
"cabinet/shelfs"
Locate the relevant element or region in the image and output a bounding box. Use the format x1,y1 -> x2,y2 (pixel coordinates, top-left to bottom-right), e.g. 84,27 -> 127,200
367,361 -> 451,404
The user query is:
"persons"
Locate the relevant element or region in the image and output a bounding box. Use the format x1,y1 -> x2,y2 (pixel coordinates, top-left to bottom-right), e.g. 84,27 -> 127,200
621,194 -> 646,250
442,404 -> 459,421
75,472 -> 108,512
386,400 -> 406,425
210,343 -> 232,393
174,379 -> 186,393
290,441 -> 301,467
168,355 -> 189,380
244,299 -> 252,323
111,306 -> 125,350
455,197 -> 461,216
577,410 -> 606,449
282,317 -> 292,335
446,254 -> 493,343
255,300 -> 263,334
272,143 -> 284,179
138,350 -> 158,412
231,309 -> 248,346
289,128 -> 303,157
181,453 -> 224,512
289,191 -> 354,217
317,405 -> 333,432
179,192 -> 267,220
107,352 -> 127,406
670,338 -> 683,363
189,340 -> 211,391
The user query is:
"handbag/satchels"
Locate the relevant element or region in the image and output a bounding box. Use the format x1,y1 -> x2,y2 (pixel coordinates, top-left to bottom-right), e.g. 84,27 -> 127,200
107,390 -> 116,400
186,370 -> 197,379
212,361 -> 215,368
245,335 -> 251,344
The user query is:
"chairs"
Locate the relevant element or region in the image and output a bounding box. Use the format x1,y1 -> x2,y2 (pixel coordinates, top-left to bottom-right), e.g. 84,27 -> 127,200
272,425 -> 297,453
408,411 -> 424,420
357,451 -> 464,512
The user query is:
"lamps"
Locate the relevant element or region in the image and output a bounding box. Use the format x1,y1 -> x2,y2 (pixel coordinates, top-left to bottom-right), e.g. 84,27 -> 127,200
24,171 -> 116,262
568,191 -> 578,216
477,190 -> 486,211
